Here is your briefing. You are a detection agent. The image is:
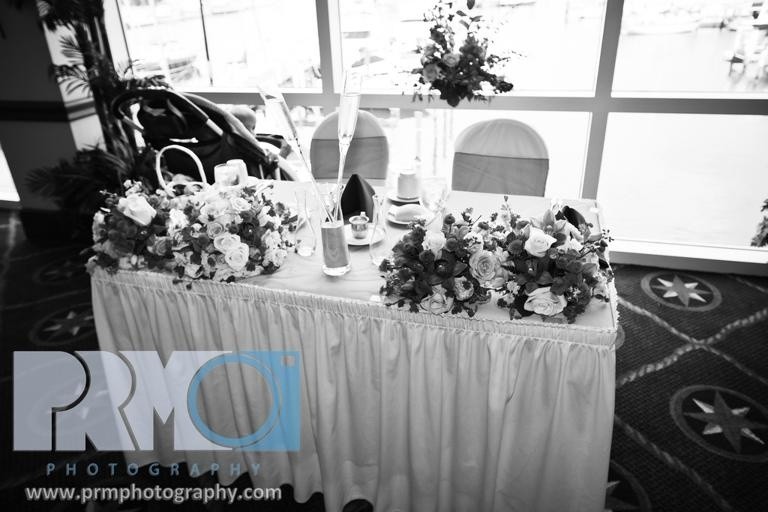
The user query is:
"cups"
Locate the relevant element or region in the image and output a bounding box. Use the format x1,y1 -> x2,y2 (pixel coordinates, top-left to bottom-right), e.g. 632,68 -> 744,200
349,211 -> 370,239
397,170 -> 420,199
213,158 -> 247,188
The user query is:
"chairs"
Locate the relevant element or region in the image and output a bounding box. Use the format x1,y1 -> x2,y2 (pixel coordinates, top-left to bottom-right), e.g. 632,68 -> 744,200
309,106 -> 390,181
452,117 -> 550,198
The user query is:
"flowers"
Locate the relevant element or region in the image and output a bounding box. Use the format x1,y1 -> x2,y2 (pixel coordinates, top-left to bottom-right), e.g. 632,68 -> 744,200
83,172 -> 304,291
379,195 -> 615,325
409,1 -> 516,109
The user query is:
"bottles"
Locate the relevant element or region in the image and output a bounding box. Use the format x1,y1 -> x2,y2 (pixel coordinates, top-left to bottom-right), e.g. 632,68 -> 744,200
292,189 -> 316,257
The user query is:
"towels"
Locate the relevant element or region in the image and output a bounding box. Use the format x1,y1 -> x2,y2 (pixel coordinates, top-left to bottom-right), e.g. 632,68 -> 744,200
326,169 -> 379,224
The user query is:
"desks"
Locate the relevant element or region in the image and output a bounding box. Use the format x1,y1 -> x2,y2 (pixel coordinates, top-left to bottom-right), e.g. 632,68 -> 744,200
94,180 -> 616,511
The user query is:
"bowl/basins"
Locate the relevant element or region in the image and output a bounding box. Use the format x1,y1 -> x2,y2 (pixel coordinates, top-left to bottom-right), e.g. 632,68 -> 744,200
395,203 -> 423,220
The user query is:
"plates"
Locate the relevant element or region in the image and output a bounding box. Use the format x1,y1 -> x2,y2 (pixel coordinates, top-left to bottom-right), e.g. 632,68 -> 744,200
388,192 -> 421,203
387,207 -> 432,226
339,223 -> 389,248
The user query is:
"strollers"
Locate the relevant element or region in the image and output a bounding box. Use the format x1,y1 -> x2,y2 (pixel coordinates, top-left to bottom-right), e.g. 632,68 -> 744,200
106,89 -> 317,188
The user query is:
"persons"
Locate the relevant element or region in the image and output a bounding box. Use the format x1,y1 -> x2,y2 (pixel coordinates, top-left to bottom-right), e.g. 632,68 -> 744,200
228,104 -> 276,161
